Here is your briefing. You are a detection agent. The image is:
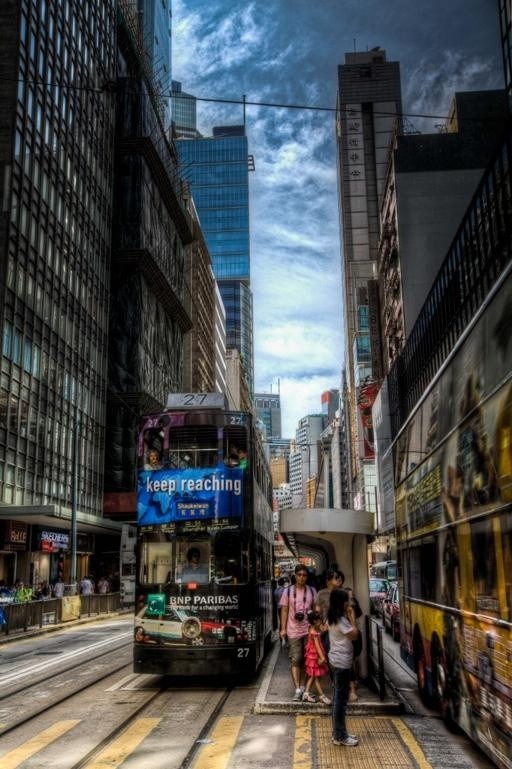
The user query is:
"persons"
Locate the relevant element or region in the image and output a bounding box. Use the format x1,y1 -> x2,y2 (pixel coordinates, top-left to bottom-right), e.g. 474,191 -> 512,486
144,449 -> 163,470
227,447 -> 247,469
1,574 -> 112,602
273,564 -> 363,746
180,547 -> 209,584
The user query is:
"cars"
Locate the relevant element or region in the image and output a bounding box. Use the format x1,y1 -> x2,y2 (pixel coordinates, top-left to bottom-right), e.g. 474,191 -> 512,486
368,579 -> 399,642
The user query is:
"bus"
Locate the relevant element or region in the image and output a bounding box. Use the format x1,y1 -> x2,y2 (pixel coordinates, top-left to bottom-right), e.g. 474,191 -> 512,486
370,560 -> 397,580
393,260 -> 512,769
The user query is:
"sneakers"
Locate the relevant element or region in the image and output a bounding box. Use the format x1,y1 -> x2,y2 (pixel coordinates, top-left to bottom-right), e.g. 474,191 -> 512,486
291,686 -> 359,704
331,734 -> 359,747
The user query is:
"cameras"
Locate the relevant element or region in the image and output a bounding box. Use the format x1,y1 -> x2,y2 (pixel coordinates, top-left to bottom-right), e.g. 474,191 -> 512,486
295,612 -> 304,620
